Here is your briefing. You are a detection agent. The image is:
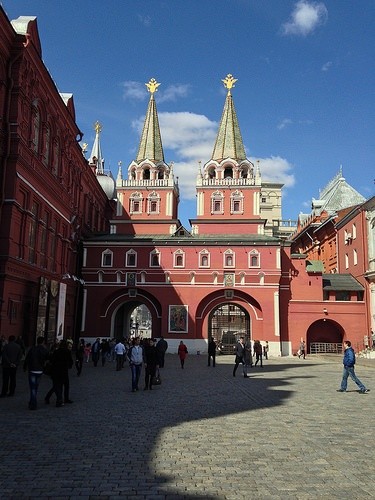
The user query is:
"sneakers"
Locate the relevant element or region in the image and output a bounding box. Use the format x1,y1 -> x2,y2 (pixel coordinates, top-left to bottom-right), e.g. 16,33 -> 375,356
55,402 -> 65,407
45,397 -> 50,405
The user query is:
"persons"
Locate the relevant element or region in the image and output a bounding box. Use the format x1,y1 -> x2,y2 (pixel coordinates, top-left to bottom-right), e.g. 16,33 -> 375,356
233,336 -> 269,378
177,341 -> 187,369
208,338 -> 216,368
336,340 -> 366,393
370,328 -> 375,347
0,334 -> 169,410
298,341 -> 306,359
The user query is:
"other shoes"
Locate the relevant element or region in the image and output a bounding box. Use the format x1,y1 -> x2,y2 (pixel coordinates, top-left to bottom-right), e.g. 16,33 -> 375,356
132,386 -> 139,392
64,399 -> 72,403
233,372 -> 236,377
244,375 -> 249,378
336,389 -> 346,392
359,387 -> 367,393
143,384 -> 148,390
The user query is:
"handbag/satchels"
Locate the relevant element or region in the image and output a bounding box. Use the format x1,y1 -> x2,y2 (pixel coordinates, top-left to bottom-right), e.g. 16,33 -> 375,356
150,367 -> 161,385
